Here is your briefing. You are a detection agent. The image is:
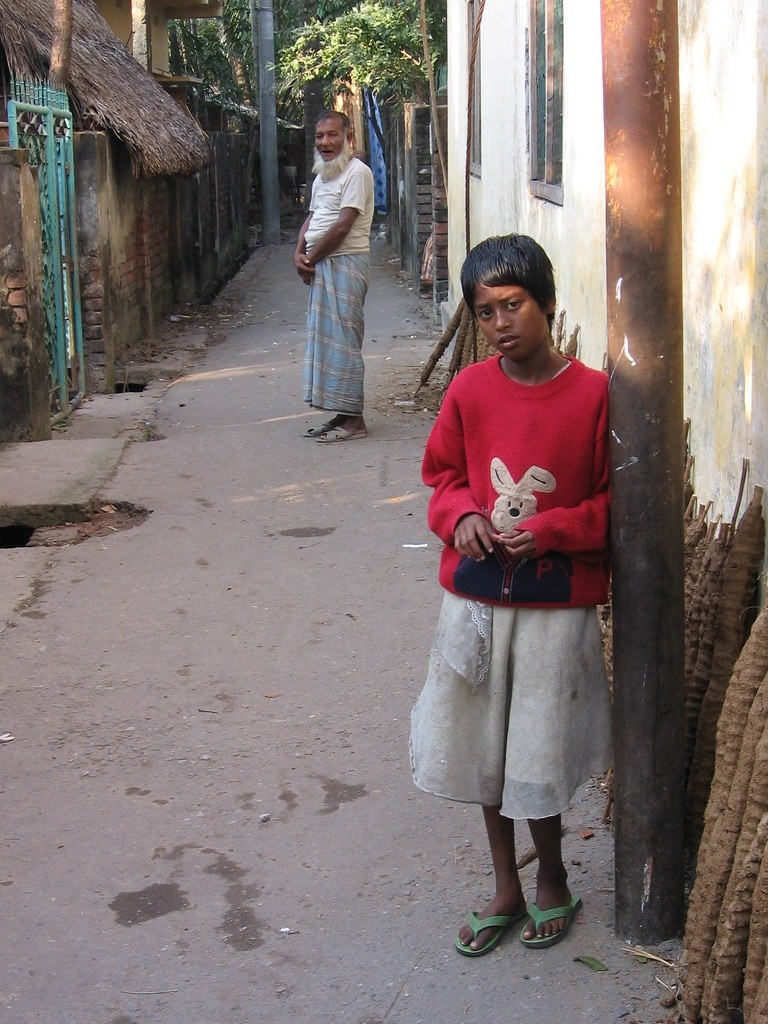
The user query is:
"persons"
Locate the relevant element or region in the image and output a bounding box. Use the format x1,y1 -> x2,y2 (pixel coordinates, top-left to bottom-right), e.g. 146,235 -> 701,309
294,111 -> 374,444
407,234 -> 610,957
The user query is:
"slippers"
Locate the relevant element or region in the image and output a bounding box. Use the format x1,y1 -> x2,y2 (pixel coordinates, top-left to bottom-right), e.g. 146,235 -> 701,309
454,901 -> 528,957
520,895 -> 582,949
304,422 -> 335,437
316,426 -> 368,442
